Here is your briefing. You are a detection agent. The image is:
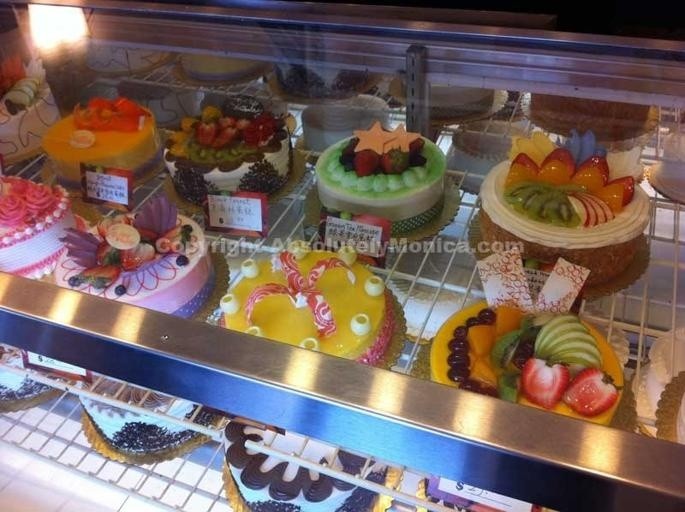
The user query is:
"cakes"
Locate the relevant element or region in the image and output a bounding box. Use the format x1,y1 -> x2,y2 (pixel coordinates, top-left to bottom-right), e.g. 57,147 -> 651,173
400,78 -> 494,118
78,396 -> 225,465
2,174 -> 89,281
42,94 -> 165,189
652,133 -> 684,198
430,299 -> 624,426
179,55 -> 265,81
55,197 -> 218,319
270,62 -> 377,100
220,240 -> 394,365
416,478 -> 546,510
0,43 -> 61,166
316,121 -> 446,233
478,129 -> 651,284
302,95 -> 390,152
163,94 -> 294,208
223,422 -> 404,512
529,92 -> 650,136
655,372 -> 684,445
1,367 -> 61,413
